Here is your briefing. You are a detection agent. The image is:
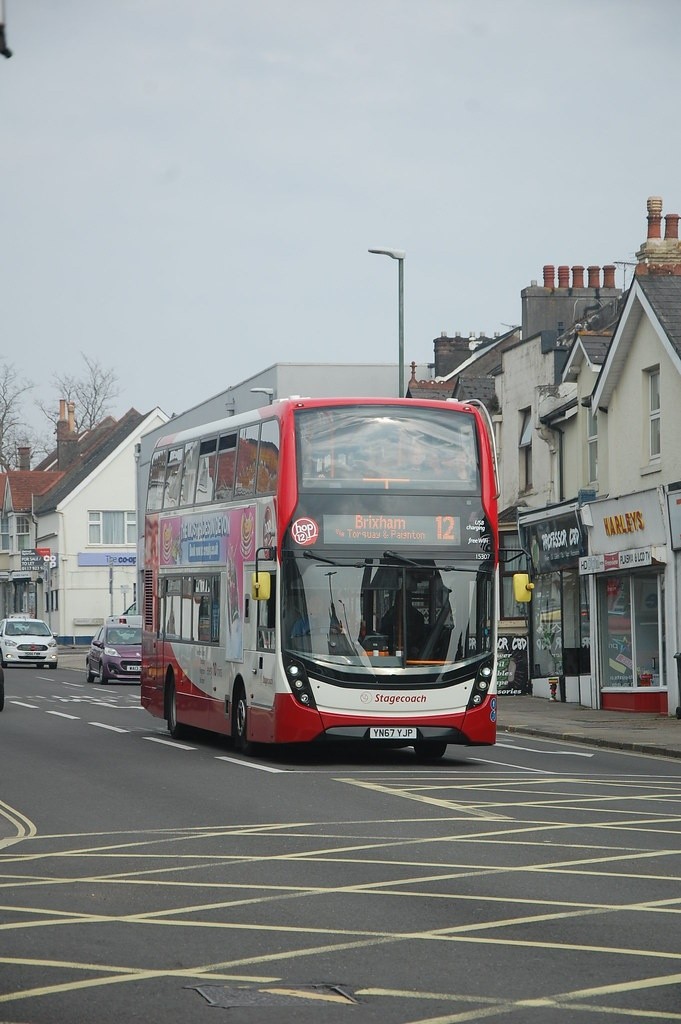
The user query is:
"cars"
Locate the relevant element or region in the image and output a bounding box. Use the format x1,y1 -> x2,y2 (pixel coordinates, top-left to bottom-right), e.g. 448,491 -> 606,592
85,623 -> 153,684
0,617 -> 59,670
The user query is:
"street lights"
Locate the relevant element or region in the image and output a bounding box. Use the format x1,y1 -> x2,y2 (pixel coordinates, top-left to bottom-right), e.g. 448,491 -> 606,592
250,387 -> 273,406
368,247 -> 404,398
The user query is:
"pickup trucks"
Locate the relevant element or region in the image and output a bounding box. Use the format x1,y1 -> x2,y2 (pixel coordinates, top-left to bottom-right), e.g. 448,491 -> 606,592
104,601 -> 139,625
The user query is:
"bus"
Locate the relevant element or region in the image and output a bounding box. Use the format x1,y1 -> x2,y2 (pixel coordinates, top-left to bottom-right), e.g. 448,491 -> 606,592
137,397 -> 502,762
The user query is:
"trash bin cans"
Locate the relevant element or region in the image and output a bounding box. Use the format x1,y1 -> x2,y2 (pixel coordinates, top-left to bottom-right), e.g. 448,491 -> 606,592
673,652 -> 681,720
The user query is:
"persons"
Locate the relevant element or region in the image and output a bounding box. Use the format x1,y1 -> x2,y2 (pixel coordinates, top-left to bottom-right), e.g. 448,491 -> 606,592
107,630 -> 126,645
380,588 -> 425,660
6,623 -> 21,634
131,631 -> 143,643
27,623 -> 44,635
287,592 -> 349,638
